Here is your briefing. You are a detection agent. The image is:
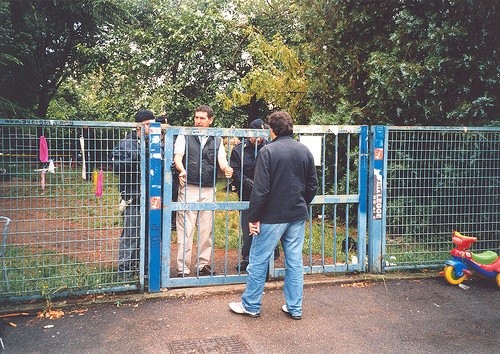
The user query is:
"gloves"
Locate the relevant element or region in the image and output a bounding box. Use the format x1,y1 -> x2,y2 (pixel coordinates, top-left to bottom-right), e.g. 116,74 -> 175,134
243,177 -> 255,190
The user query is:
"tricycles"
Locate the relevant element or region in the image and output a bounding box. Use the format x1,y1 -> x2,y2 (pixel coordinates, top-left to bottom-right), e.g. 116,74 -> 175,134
438,230 -> 500,289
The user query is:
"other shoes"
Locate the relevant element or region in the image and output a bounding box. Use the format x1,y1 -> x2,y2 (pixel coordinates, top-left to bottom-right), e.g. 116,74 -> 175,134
228,302 -> 261,318
237,261 -> 249,270
200,265 -> 215,275
281,304 -> 302,320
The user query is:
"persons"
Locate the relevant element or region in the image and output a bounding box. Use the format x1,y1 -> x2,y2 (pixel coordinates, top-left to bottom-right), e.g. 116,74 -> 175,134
230,118 -> 279,271
111,110 -> 176,278
173,105 -> 234,278
228,111 -> 319,321
156,116 -> 178,231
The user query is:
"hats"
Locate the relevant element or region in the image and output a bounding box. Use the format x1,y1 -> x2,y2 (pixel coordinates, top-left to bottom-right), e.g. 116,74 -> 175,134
249,118 -> 267,129
156,116 -> 170,123
134,110 -> 155,122
